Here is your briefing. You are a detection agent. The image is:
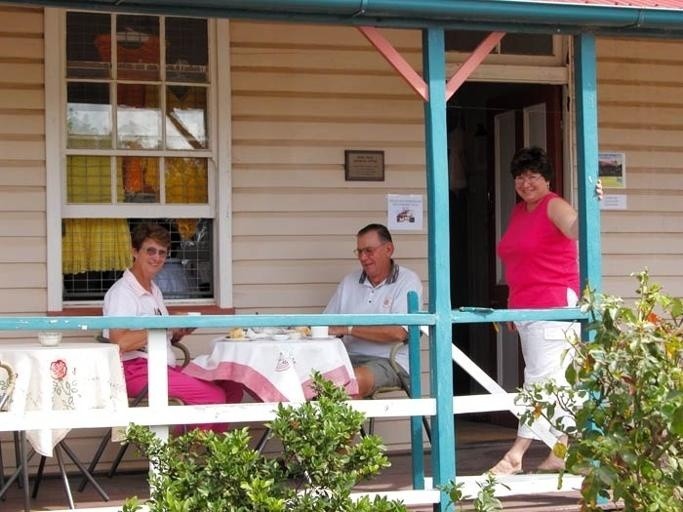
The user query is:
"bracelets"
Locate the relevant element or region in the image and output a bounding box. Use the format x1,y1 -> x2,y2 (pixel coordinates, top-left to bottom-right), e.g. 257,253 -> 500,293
348,325 -> 353,336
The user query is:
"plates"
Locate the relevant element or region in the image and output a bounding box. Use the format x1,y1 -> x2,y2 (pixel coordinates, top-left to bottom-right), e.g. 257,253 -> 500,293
303,335 -> 337,340
222,336 -> 257,342
270,334 -> 291,341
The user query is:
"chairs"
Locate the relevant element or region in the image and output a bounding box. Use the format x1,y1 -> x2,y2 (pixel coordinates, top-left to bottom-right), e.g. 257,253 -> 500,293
77,310 -> 239,497
354,332 -> 430,472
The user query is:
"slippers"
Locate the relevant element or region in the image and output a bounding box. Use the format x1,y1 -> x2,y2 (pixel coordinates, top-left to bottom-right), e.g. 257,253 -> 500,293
482,460 -> 524,475
535,459 -> 567,472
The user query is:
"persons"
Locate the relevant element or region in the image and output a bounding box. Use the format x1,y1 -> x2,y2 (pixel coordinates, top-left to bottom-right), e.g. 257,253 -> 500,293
97,220 -> 244,471
304,222 -> 426,470
149,222 -> 201,302
474,146 -> 604,478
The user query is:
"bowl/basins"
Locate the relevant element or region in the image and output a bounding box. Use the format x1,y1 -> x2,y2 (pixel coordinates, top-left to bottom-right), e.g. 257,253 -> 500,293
309,326 -> 329,338
38,331 -> 63,346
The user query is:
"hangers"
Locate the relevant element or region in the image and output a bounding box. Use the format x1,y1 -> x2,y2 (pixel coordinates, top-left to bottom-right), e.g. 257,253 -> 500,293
111,22 -> 150,43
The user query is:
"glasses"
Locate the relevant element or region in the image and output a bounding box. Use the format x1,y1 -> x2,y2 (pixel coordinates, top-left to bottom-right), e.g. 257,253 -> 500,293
515,175 -> 542,184
143,247 -> 170,258
353,243 -> 384,255
